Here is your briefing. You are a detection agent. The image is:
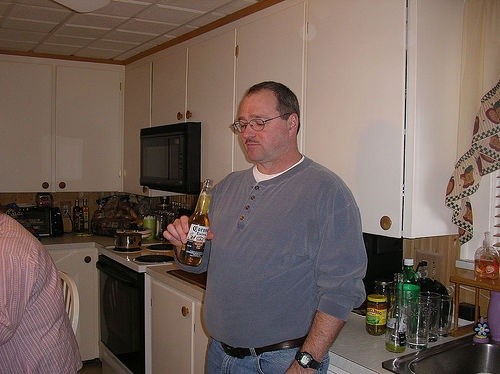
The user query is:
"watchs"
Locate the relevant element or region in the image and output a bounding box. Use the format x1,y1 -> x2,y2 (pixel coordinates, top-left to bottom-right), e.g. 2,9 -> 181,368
295,351 -> 321,370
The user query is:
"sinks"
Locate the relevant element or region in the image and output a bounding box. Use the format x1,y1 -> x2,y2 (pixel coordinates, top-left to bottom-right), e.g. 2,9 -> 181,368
382,334 -> 500,374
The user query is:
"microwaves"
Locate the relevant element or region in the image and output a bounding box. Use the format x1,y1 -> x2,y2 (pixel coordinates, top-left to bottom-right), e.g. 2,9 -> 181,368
140,121 -> 201,195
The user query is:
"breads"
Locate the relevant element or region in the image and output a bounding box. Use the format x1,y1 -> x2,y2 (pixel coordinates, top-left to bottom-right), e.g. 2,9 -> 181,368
474,255 -> 500,280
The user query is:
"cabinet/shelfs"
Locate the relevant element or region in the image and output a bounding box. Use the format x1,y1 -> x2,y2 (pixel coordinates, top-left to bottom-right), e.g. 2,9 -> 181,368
125,46 -> 152,197
43,242 -> 100,363
0,48 -> 123,192
151,11 -> 236,188
151,280 -> 206,374
233,0 -> 465,239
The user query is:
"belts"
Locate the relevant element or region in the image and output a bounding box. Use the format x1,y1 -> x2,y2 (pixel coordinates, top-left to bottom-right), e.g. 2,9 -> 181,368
221,334 -> 308,359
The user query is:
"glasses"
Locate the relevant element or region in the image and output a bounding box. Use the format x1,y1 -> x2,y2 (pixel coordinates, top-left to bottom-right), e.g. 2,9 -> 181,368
233,112 -> 291,133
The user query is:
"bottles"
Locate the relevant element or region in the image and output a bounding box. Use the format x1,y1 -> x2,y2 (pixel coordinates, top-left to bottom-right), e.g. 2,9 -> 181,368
154,196 -> 170,242
81,199 -> 89,233
396,259 -> 421,329
177,178 -> 214,267
488,290 -> 500,344
72,199 -> 83,233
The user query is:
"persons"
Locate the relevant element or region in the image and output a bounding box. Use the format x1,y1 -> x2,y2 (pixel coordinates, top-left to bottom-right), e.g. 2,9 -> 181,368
0,211 -> 84,374
163,82 -> 366,374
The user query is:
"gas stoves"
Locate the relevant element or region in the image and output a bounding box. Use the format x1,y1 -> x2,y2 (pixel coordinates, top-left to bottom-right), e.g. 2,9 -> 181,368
102,242 -> 177,273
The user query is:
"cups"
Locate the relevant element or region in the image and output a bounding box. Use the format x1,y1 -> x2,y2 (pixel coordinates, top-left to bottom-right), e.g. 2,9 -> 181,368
385,292 -> 453,354
374,279 -> 394,296
142,216 -> 155,240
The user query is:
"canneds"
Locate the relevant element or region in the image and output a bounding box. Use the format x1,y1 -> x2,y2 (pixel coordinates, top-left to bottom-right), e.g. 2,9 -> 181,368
367,295 -> 387,335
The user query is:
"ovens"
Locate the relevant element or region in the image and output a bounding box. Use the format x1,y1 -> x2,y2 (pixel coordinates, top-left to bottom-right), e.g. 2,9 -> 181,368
0,207 -> 63,238
95,255 -> 145,374
